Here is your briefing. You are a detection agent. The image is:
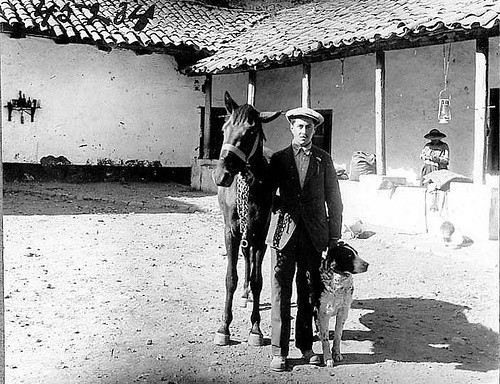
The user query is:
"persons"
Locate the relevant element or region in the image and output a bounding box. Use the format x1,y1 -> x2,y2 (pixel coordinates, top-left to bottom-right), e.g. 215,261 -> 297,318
269,107 -> 342,371
418,129 -> 450,186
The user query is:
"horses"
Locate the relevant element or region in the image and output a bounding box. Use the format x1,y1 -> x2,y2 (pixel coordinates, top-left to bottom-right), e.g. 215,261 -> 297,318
210,90 -> 276,346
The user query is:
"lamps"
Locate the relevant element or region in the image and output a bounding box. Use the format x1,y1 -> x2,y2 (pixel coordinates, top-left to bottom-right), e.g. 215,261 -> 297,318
436,43 -> 453,124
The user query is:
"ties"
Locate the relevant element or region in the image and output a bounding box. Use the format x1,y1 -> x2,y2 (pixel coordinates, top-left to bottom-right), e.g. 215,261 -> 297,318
298,150 -> 305,184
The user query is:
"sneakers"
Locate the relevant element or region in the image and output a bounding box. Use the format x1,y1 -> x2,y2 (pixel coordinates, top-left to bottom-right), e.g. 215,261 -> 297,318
302,350 -> 322,364
270,356 -> 288,372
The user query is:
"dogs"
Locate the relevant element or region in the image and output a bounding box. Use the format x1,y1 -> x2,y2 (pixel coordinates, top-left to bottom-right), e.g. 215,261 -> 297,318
306,242 -> 369,368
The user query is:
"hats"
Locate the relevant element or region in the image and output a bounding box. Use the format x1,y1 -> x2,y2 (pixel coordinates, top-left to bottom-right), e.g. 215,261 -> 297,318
285,107 -> 325,129
423,129 -> 447,140
